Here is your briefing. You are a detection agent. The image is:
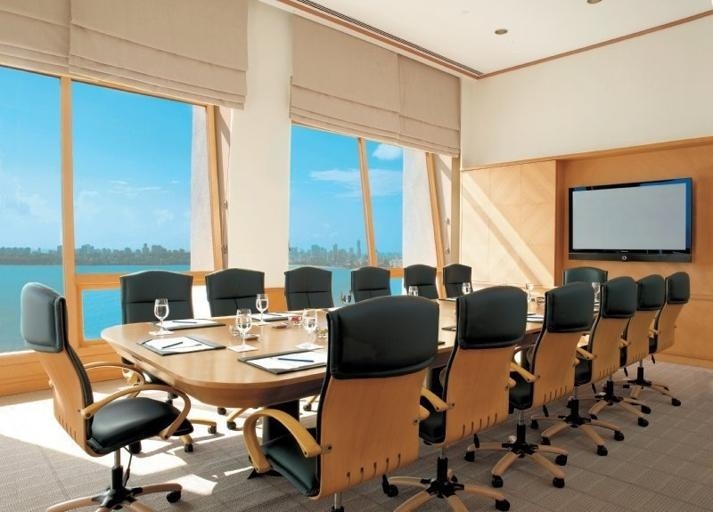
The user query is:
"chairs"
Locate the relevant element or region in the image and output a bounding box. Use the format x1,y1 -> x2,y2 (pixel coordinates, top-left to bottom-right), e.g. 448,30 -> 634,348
21,283 -> 191,512
578,274 -> 665,427
244,295 -> 440,512
529,276 -> 637,456
206,268 -> 268,429
443,264 -> 473,298
464,283 -> 594,488
351,266 -> 390,303
120,270 -> 216,454
285,267 -> 334,412
382,286 -> 527,512
404,265 -> 438,299
562,267 -> 608,286
613,272 -> 690,406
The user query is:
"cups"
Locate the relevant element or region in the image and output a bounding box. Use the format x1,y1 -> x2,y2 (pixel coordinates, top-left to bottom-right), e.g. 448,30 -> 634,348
525,280 -> 533,304
591,282 -> 600,305
340,290 -> 352,306
153,298 -> 170,337
236,308 -> 252,350
255,294 -> 269,325
461,282 -> 471,295
408,286 -> 418,297
302,308 -> 317,349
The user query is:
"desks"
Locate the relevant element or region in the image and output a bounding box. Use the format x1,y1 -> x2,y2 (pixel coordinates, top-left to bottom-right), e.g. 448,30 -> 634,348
101,300 -> 599,479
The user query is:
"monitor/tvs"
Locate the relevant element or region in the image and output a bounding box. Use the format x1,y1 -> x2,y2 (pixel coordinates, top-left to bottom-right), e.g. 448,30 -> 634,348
568,177 -> 694,263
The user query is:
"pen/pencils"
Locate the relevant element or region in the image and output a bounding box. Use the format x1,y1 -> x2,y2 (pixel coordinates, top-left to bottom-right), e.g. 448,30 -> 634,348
172,320 -> 197,323
278,358 -> 314,362
267,313 -> 282,316
527,316 -> 545,319
162,342 -> 183,348
